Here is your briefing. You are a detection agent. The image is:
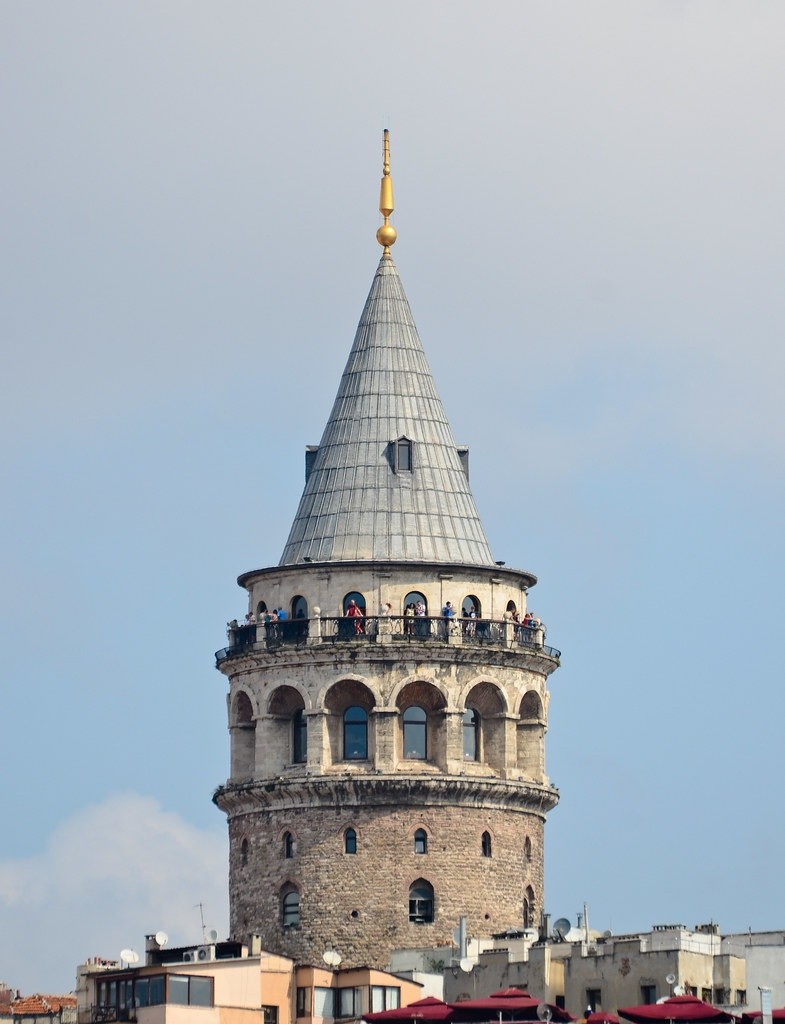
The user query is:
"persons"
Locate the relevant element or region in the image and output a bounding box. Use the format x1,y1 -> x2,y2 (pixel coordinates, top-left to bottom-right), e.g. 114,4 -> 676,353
345,599 -> 364,636
467,605 -> 479,636
379,599 -> 456,634
245,607 -> 286,638
508,608 -> 538,643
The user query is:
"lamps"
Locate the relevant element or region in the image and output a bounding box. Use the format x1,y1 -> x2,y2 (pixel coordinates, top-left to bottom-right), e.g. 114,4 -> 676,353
495,561 -> 505,565
303,556 -> 313,562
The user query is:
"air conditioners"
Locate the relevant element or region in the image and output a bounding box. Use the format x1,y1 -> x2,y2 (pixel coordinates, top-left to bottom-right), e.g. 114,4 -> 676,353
183,950 -> 198,962
197,944 -> 217,961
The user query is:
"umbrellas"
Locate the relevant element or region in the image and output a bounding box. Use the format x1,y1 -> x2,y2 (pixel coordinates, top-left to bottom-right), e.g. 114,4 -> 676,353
446,987 -> 576,1024
617,996 -> 743,1024
588,1012 -> 620,1024
742,1008 -> 785,1024
362,996 -> 500,1024
